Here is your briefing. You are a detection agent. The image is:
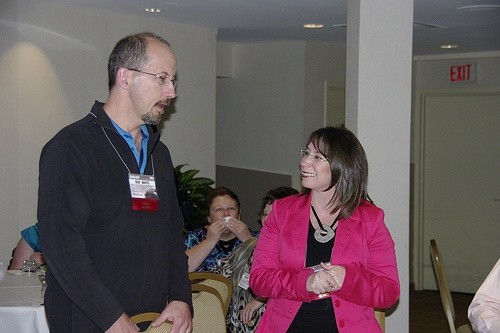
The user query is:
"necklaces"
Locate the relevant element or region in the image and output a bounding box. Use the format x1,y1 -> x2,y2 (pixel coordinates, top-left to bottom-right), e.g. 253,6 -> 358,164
309,204 -> 339,243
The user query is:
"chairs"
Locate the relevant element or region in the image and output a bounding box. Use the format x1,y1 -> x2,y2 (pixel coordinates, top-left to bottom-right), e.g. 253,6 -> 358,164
130,272 -> 233,333
429,239 -> 476,333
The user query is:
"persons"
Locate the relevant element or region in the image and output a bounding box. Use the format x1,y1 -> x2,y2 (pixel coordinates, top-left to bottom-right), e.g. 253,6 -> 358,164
37,32 -> 193,333
211,187 -> 301,333
184,187 -> 257,274
249,127 -> 400,333
9,223 -> 46,270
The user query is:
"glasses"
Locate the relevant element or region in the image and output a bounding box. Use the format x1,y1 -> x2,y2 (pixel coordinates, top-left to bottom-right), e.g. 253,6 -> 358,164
123,66 -> 178,89
209,205 -> 238,215
299,149 -> 328,163
261,211 -> 269,219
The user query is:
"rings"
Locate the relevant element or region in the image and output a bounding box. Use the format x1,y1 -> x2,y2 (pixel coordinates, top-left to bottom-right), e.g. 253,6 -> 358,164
186,329 -> 192,333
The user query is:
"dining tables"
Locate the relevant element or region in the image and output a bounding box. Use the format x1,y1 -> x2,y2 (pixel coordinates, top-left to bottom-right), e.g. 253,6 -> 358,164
0,269 -> 50,333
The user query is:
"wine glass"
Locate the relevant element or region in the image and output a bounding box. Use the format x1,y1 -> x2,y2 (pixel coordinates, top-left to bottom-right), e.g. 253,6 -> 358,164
23,258 -> 36,279
0,261 -> 7,281
38,269 -> 47,305
